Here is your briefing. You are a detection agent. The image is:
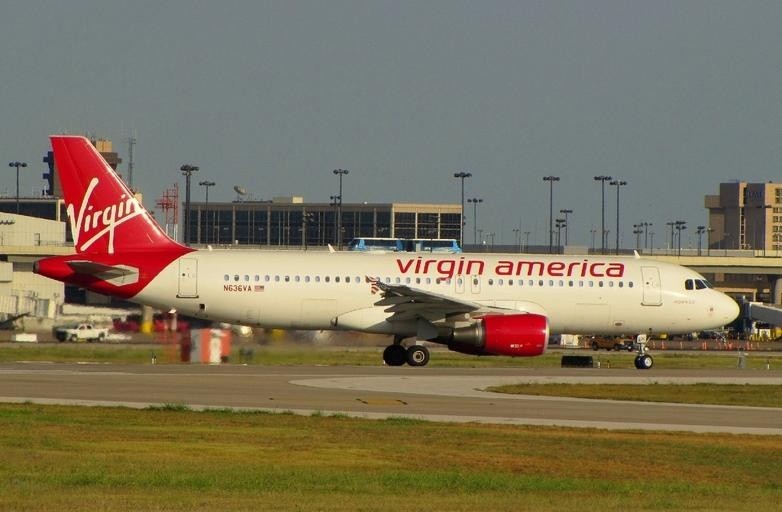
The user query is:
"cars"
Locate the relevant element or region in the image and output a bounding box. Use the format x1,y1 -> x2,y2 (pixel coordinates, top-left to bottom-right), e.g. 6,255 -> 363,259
592,336 -> 633,352
698,329 -> 724,343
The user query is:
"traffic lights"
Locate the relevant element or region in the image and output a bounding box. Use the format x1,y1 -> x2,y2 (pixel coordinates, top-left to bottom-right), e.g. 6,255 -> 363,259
43,152 -> 53,195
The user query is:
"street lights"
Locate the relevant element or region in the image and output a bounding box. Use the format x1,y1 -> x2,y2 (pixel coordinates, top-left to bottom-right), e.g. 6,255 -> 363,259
543,176 -> 716,256
180,165 -> 215,246
329,168 -> 349,250
9,161 -> 27,214
453,172 -> 483,250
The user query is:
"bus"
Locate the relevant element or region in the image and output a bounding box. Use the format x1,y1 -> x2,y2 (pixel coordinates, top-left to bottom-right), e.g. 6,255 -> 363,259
349,237 -> 462,254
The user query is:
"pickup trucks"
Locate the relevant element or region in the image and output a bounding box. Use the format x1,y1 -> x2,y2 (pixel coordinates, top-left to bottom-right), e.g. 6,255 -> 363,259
56,323 -> 108,342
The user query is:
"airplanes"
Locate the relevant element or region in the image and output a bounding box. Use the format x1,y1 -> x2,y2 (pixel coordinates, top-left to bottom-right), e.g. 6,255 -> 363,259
32,135 -> 740,369
